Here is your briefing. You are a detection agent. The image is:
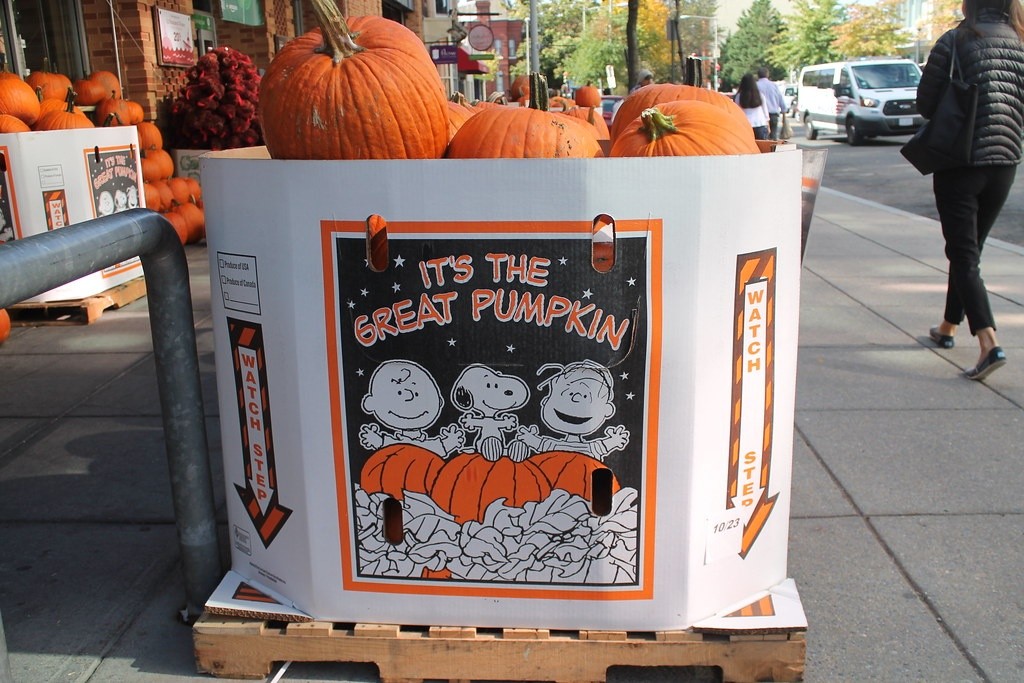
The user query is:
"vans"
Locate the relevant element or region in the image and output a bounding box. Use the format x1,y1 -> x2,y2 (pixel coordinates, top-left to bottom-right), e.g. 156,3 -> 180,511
796,59 -> 928,146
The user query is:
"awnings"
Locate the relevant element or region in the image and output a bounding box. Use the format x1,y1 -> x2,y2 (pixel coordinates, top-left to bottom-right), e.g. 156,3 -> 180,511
458,47 -> 489,74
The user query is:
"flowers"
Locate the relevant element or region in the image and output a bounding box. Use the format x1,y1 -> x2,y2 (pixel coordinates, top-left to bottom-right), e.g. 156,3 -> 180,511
169,46 -> 263,151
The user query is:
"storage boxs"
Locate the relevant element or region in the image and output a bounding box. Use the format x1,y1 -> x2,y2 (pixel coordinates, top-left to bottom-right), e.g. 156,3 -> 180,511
196,141 -> 803,633
0,126 -> 144,302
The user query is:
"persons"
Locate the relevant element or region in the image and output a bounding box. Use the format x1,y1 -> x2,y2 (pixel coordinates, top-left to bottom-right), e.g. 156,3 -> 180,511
915,0 -> 1024,378
756,67 -> 788,152
733,73 -> 771,140
887,67 -> 899,83
630,69 -> 652,94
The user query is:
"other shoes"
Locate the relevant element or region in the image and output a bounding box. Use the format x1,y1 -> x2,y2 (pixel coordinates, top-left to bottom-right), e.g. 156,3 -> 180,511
929,326 -> 954,348
965,346 -> 1007,380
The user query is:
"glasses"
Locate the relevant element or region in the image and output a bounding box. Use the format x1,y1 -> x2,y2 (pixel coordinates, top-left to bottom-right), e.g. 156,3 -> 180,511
645,75 -> 654,80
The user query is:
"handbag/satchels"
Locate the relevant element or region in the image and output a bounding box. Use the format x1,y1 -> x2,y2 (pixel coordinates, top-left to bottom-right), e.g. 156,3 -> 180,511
779,119 -> 793,140
900,29 -> 979,177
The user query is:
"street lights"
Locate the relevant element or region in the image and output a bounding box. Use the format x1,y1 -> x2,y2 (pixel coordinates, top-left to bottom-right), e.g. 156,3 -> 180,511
580,1 -> 628,31
678,15 -> 718,93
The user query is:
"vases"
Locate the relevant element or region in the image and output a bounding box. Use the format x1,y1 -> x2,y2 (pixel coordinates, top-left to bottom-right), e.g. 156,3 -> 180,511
170,149 -> 207,246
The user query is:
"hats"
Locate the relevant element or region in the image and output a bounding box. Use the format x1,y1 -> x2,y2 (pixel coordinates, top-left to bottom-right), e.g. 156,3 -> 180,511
636,69 -> 653,85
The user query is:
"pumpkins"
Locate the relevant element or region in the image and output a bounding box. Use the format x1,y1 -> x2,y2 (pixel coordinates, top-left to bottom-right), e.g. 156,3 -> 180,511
254,0 -> 766,159
0,56 -> 206,245
0,308 -> 11,344
